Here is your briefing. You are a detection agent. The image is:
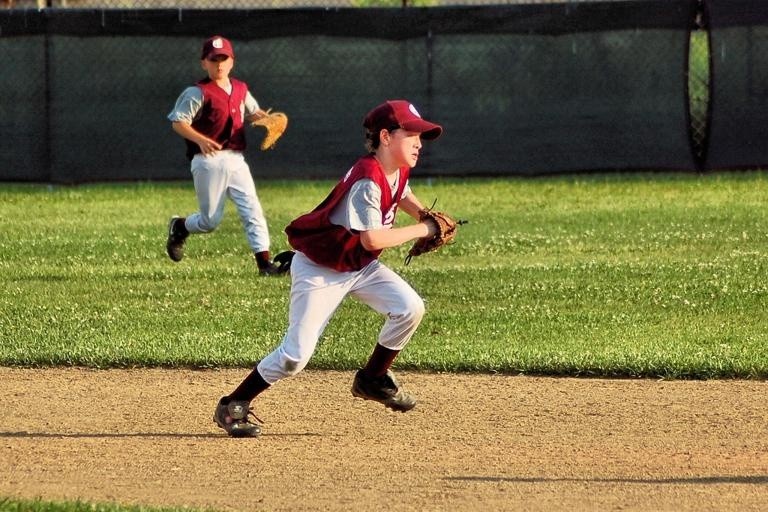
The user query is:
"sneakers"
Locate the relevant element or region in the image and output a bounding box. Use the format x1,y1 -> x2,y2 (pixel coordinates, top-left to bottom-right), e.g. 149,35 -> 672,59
257,262 -> 286,277
211,396 -> 266,438
350,366 -> 417,414
164,213 -> 187,262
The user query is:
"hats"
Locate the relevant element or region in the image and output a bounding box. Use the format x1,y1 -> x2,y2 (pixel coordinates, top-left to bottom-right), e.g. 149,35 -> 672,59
361,97 -> 442,143
201,34 -> 235,61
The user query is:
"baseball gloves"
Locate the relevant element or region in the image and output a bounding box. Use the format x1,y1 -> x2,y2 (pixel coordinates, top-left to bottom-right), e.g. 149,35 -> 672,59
404,198 -> 468,266
245,108 -> 288,151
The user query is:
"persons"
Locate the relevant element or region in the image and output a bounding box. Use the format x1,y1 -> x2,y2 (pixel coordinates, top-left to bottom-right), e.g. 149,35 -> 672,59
212,98 -> 457,437
164,34 -> 297,275
